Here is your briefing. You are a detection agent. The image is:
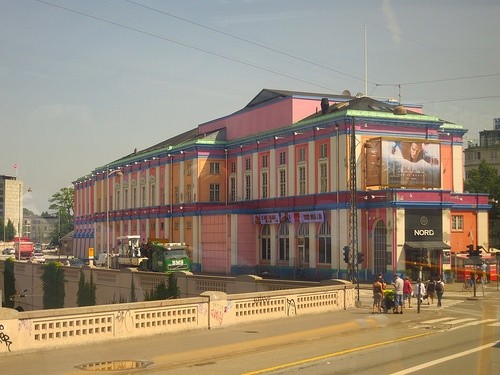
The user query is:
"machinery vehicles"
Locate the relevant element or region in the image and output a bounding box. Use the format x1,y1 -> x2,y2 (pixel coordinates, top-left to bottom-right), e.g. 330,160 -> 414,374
109,235 -> 148,271
138,240 -> 192,274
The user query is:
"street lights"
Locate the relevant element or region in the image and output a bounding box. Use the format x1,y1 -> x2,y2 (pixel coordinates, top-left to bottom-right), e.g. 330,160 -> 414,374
105,165 -> 123,269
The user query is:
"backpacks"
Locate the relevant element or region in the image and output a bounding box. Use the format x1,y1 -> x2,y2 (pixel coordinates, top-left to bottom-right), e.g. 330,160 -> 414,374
436,281 -> 442,291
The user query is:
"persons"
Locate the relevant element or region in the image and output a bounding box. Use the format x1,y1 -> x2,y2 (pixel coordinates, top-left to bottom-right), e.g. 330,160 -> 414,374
436,278 -> 444,307
426,277 -> 435,305
372,272 -> 413,314
469,272 -> 474,293
414,278 -> 425,305
392,141 -> 439,165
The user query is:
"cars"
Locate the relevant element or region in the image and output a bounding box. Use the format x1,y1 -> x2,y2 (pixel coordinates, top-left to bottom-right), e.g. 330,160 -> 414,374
35,243 -> 57,253
34,248 -> 44,256
29,253 -> 46,263
2,248 -> 15,255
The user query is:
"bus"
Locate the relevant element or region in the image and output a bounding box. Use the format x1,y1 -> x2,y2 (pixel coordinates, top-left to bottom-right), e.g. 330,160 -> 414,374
14,242 -> 33,260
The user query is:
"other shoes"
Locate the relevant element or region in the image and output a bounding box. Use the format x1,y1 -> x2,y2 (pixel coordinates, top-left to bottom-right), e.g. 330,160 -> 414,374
399,311 -> 403,314
394,311 -> 400,314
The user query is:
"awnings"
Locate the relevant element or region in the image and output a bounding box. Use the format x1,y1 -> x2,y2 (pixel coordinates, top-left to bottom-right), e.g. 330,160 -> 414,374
404,241 -> 451,251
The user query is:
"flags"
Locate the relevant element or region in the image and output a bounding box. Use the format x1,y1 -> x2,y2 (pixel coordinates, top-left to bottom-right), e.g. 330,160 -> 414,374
12,162 -> 17,168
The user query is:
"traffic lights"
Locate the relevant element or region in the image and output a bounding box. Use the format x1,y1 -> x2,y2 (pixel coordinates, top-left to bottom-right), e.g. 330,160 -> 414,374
357,251 -> 364,264
477,246 -> 483,265
466,244 -> 473,258
343,246 -> 349,263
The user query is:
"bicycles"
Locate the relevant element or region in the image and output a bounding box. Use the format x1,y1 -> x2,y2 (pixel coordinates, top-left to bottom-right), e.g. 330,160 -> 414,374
463,279 -> 473,294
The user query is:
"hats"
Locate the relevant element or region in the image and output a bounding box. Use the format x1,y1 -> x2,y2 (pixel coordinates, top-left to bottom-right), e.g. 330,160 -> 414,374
378,272 -> 382,276
